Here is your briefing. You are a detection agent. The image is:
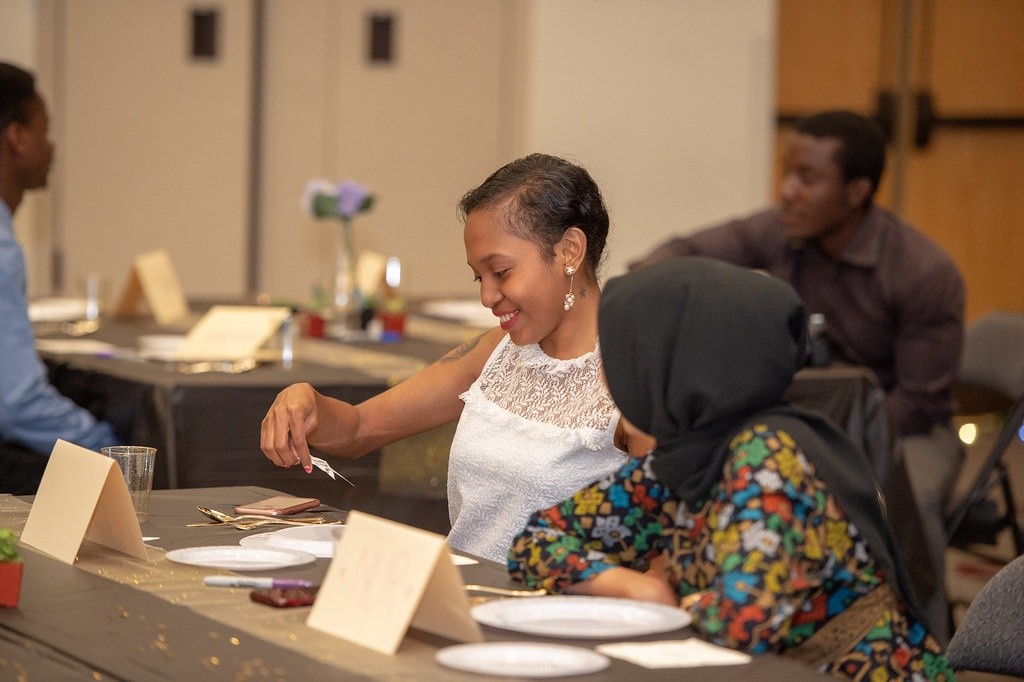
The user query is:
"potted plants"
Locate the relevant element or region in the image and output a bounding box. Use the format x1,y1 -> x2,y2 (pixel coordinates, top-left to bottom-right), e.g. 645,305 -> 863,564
0,529 -> 25,609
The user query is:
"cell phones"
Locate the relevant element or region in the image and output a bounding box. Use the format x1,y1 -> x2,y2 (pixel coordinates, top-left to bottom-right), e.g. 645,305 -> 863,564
251,586 -> 322,609
234,496 -> 320,518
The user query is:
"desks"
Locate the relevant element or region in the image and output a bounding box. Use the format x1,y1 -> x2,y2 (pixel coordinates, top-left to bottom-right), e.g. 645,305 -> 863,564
32,290 -> 493,536
0,483 -> 838,682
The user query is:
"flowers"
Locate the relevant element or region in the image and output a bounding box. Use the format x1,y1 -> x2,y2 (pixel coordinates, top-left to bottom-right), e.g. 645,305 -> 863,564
303,175 -> 380,292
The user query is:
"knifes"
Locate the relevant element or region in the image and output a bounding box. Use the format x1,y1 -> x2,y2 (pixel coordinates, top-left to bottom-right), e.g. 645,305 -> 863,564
233,506 -> 337,518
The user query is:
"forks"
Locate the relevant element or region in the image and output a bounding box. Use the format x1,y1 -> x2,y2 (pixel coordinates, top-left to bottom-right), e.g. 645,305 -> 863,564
230,520 -> 344,531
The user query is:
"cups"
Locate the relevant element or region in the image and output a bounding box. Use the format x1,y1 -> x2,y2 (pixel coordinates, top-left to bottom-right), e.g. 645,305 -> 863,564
100,445 -> 157,524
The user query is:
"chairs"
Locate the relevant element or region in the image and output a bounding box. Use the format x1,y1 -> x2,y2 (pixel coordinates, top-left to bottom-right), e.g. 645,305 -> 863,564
944,309 -> 1024,560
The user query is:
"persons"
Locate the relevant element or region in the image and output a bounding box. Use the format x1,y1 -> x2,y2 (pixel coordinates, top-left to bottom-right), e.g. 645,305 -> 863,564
626,110 -> 969,573
506,254 -> 954,682
1,61 -> 119,496
259,153 -> 656,566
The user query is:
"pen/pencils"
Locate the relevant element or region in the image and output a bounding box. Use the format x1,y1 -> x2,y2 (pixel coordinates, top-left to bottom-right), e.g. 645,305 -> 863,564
203,575 -> 315,596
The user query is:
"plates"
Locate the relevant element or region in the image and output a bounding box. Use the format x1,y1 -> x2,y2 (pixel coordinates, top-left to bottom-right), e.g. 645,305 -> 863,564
166,545 -> 315,573
468,594 -> 694,641
435,641 -> 611,680
238,524 -> 353,559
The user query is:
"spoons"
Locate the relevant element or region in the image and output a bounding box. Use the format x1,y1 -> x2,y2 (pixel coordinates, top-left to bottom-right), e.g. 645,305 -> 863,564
196,499 -> 320,523
